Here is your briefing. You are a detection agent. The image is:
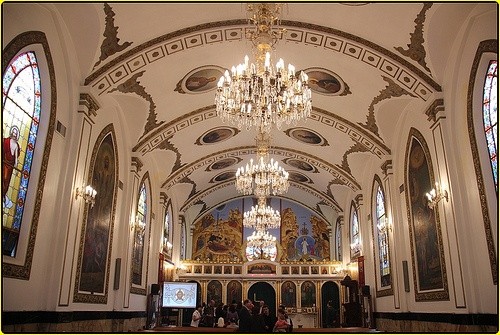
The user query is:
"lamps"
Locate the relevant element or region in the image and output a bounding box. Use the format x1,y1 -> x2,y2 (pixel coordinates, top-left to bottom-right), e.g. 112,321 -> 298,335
234,129 -> 290,196
377,221 -> 392,233
243,198 -> 281,229
75,185 -> 97,207
427,188 -> 448,208
131,220 -> 146,235
214,3 -> 312,129
246,228 -> 277,261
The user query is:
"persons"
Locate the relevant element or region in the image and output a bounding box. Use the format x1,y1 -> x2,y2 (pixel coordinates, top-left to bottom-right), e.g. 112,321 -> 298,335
273,305 -> 293,332
190,300 -> 239,328
238,299 -> 274,332
